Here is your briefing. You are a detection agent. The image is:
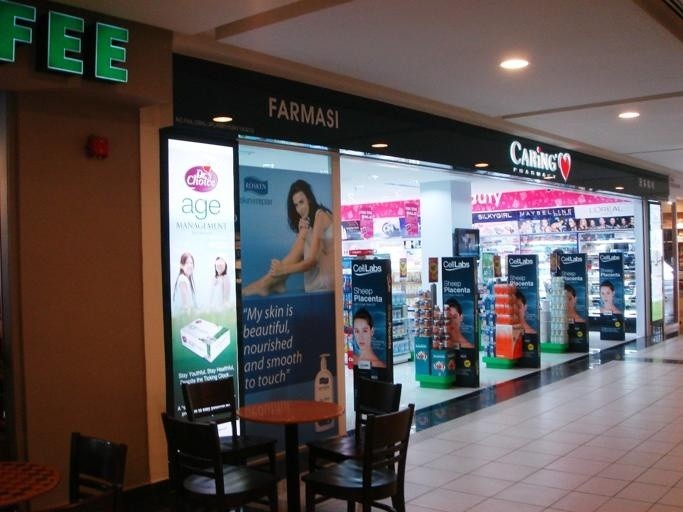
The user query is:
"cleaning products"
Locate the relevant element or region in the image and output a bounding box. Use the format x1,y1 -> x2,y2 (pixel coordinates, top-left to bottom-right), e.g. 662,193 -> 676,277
314,352 -> 336,434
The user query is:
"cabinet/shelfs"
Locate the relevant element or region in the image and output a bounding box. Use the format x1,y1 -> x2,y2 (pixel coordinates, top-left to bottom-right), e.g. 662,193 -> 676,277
479,230 -> 636,333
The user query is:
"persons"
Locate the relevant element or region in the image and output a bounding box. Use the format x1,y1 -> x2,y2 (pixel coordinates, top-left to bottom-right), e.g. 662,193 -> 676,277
240,178 -> 334,297
460,233 -> 474,249
519,215 -> 635,234
516,290 -> 536,334
446,298 -> 473,349
565,286 -> 585,323
210,255 -> 230,311
599,281 -> 620,314
172,253 -> 196,313
350,307 -> 386,367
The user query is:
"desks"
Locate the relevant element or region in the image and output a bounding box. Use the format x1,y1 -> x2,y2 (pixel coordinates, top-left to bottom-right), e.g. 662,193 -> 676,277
0,462 -> 61,512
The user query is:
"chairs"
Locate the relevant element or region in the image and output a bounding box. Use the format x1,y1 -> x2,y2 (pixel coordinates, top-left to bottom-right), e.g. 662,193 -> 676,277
67,432 -> 129,511
160,378 -> 415,512
